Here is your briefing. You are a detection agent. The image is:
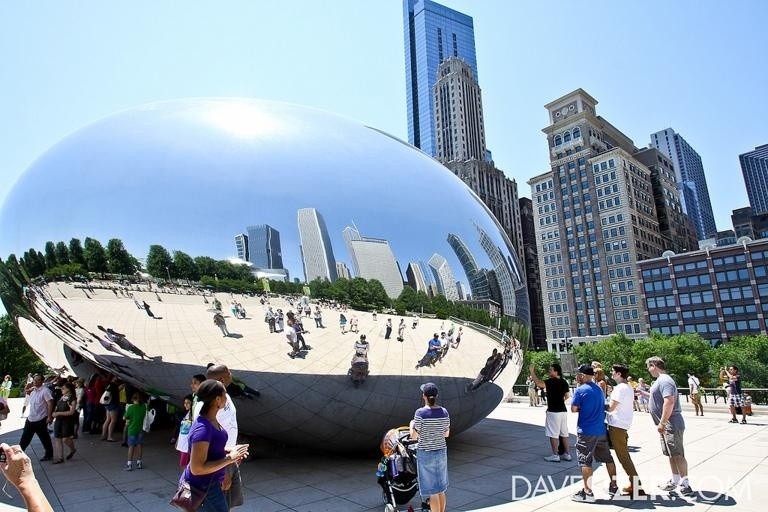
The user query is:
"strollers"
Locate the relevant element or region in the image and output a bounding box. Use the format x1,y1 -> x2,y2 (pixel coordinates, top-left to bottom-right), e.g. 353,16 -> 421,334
345,352 -> 372,389
374,424 -> 420,512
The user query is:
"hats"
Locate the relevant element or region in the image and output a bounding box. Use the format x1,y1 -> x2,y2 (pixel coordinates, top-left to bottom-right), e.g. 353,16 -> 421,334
578,364 -> 596,376
420,382 -> 439,396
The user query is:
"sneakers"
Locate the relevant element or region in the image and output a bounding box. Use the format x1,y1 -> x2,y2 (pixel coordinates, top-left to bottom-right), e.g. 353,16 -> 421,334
668,483 -> 695,497
66,449 -> 77,460
570,487 -> 596,503
559,452 -> 572,461
604,482 -> 619,502
657,478 -> 677,491
40,456 -> 54,461
621,485 -> 644,495
52,458 -> 64,465
123,464 -> 132,471
544,454 -> 561,462
135,462 -> 143,469
730,418 -> 747,424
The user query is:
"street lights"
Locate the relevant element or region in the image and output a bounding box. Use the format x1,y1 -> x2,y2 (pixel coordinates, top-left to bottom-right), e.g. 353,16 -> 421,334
107,259 -> 114,280
560,335 -> 573,353
304,283 -> 308,296
263,279 -> 270,304
165,266 -> 171,282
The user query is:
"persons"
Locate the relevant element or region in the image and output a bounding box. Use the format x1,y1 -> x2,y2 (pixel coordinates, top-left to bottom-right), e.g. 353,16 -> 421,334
24,277 -> 519,392
683,369 -> 705,416
720,364 -> 746,424
1,367 -> 251,512
526,356 -> 690,502
410,382 -> 451,509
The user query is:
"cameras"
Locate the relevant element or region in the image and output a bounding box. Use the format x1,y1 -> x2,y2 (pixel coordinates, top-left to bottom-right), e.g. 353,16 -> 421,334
0,449 -> 9,464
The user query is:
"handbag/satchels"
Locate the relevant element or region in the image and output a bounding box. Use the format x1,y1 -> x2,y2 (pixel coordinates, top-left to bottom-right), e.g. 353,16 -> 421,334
168,478 -> 209,512
143,408 -> 156,433
